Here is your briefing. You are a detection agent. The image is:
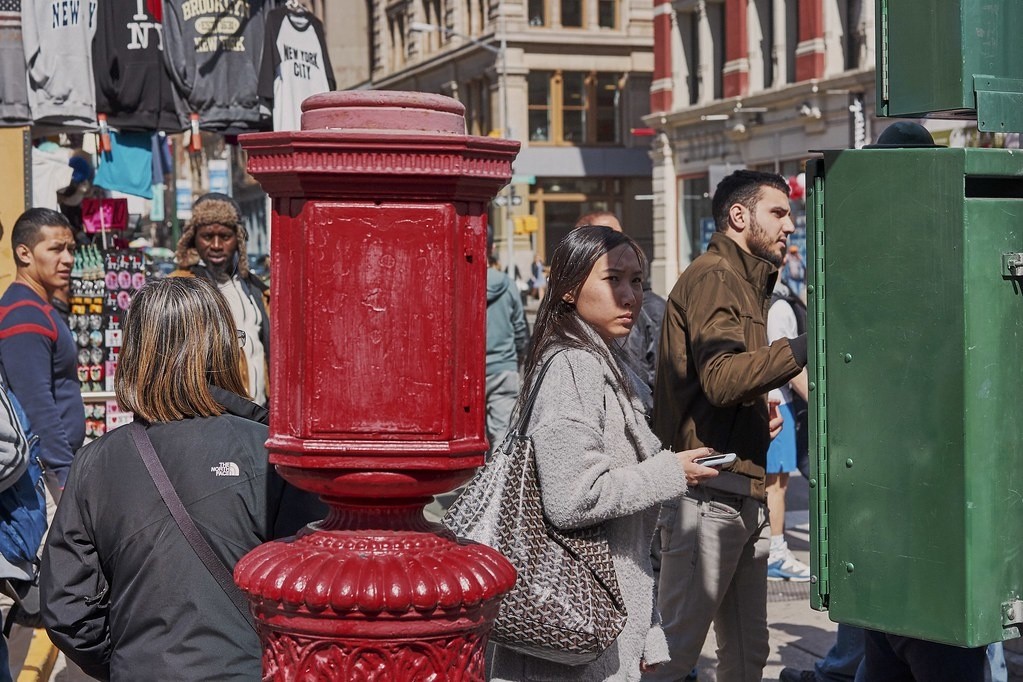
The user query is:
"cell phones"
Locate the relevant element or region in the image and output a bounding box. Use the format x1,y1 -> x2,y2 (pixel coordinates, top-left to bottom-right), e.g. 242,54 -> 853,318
693,453 -> 737,467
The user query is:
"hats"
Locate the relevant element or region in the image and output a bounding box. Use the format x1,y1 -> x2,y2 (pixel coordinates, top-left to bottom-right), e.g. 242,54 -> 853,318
175,192 -> 249,280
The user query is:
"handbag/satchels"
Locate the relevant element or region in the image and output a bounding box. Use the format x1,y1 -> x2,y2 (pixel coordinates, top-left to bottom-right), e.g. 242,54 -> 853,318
440,348 -> 628,665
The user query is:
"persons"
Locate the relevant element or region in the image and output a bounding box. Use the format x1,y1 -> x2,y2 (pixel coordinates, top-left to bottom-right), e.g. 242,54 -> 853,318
0,169 -> 1008,681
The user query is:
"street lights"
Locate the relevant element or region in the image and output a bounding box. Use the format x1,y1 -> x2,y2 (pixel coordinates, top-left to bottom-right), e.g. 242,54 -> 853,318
407,21 -> 517,263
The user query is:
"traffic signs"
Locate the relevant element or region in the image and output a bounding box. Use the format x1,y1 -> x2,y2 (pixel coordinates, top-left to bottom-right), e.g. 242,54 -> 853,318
493,194 -> 522,206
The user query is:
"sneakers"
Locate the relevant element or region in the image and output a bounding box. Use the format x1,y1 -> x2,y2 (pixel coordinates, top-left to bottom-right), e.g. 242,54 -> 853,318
767,540 -> 811,583
